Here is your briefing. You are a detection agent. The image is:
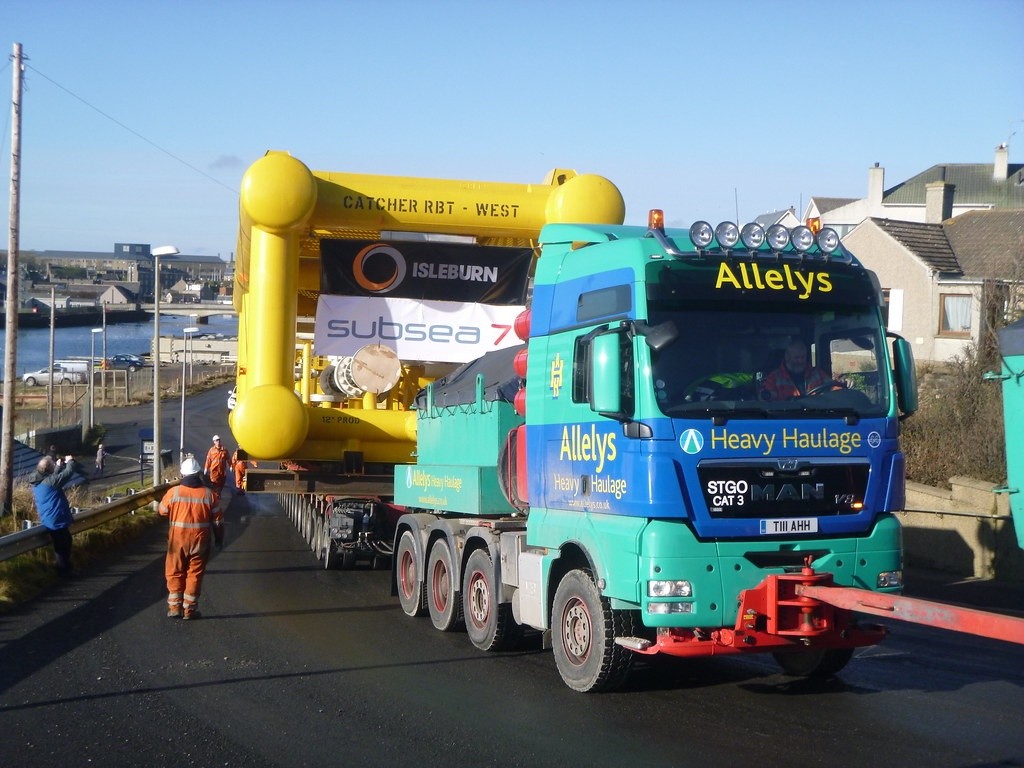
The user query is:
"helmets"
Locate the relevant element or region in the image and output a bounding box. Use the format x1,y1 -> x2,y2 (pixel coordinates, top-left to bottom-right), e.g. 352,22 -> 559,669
180,458 -> 201,475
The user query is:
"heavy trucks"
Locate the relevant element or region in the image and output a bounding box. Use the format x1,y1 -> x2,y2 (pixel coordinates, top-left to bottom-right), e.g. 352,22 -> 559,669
273,207 -> 919,695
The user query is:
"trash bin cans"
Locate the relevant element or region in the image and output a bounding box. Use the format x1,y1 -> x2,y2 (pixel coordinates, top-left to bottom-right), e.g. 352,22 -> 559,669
160,450 -> 174,469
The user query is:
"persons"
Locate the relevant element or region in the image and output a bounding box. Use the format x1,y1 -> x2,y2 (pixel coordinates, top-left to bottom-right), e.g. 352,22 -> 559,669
765,337 -> 856,400
158,454 -> 224,620
29,434 -> 258,570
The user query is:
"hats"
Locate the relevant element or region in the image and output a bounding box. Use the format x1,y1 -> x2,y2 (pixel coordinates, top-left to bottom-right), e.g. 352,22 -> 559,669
212,435 -> 221,442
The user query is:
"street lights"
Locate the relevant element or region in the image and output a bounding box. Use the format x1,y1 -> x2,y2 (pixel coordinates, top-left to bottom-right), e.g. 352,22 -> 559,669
151,245 -> 180,489
189,314 -> 199,386
88,327 -> 105,427
180,327 -> 201,464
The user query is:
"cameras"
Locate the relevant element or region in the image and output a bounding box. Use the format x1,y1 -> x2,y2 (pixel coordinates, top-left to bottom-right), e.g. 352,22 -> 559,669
60,457 -> 65,462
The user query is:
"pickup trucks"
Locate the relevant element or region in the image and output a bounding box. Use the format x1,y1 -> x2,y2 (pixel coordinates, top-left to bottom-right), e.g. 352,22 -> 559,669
21,367 -> 85,386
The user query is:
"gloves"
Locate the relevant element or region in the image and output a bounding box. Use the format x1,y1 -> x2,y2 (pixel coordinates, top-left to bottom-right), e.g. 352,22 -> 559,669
204,469 -> 207,475
230,467 -> 234,471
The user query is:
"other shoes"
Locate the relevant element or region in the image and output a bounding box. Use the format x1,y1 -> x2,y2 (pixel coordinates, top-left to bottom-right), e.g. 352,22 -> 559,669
167,610 -> 180,617
57,570 -> 82,581
183,611 -> 200,619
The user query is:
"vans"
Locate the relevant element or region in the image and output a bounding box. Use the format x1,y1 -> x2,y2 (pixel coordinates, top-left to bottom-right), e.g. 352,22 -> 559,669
49,361 -> 91,381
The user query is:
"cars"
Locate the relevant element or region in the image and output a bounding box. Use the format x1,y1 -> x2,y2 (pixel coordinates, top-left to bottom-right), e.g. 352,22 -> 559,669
101,352 -> 145,373
227,386 -> 239,410
